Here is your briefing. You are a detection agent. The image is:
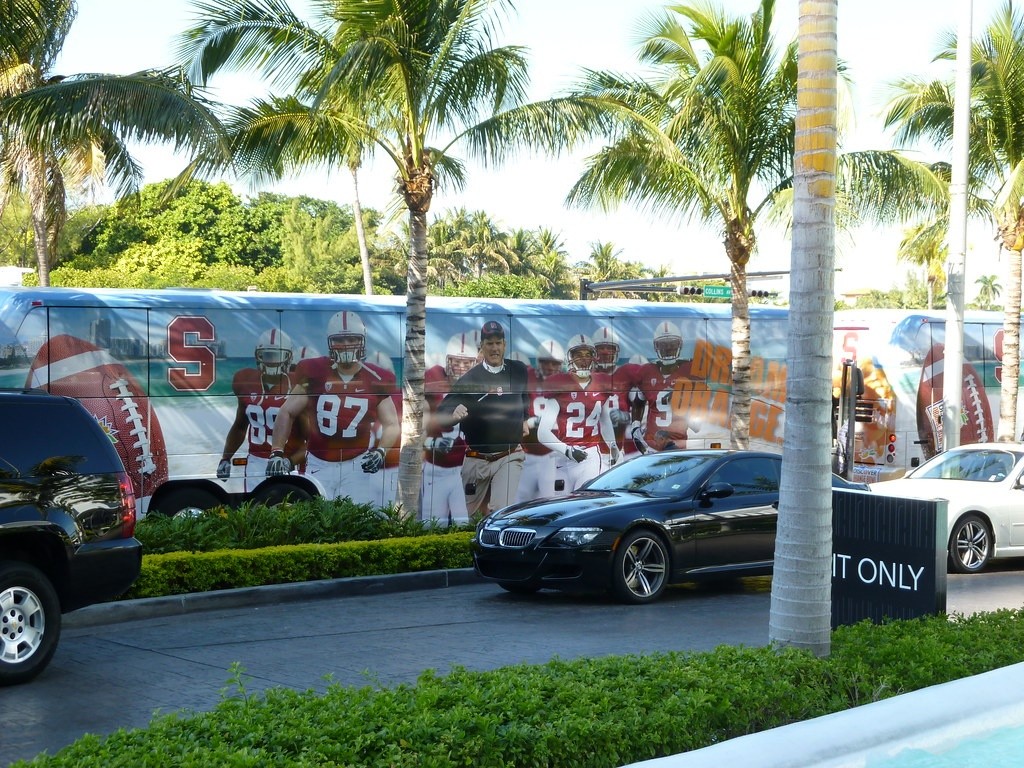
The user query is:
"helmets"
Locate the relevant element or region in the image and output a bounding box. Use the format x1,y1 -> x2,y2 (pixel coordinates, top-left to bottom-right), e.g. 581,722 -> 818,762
446,333 -> 478,381
506,352 -> 531,366
593,327 -> 619,371
537,340 -> 564,379
566,334 -> 596,378
654,322 -> 683,365
327,311 -> 365,369
255,329 -> 291,375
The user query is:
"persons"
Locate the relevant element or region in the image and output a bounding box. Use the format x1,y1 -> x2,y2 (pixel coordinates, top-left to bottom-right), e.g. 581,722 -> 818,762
435,321 -> 530,512
216,328 -> 307,492
290,346 -> 321,369
470,329 -> 484,364
265,311 -> 400,503
365,350 -> 403,502
593,327 -> 648,472
628,321 -> 702,453
507,339 -> 564,501
537,334 -> 623,495
422,333 -> 477,527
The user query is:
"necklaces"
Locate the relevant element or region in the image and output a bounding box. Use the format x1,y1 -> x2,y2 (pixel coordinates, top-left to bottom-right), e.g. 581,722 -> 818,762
485,360 -> 502,373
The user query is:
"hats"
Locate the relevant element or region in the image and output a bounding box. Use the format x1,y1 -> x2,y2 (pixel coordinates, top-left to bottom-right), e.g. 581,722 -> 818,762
481,321 -> 504,341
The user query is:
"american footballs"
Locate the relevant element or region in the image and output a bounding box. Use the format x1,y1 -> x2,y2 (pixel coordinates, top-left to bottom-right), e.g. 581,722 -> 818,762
24,333 -> 169,522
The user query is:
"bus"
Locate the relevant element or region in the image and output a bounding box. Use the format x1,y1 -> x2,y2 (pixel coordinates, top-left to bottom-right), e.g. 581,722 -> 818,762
0,287 -> 866,537
835,309 -> 1024,482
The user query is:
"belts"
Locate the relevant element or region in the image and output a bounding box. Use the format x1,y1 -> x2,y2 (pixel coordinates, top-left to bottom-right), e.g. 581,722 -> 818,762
466,448 -> 521,462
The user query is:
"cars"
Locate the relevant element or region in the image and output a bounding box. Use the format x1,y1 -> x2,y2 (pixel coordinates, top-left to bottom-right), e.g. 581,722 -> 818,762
868,443 -> 1024,577
469,449 -> 871,604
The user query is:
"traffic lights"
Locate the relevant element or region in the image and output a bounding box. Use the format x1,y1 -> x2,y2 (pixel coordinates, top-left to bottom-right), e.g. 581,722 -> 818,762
679,287 -> 704,296
748,289 -> 770,298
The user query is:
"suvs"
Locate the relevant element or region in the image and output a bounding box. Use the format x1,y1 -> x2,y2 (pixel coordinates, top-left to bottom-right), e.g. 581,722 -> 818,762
1,387 -> 145,679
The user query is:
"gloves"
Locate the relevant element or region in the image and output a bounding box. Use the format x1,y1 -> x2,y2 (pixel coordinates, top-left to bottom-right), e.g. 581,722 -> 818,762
431,437 -> 453,454
361,449 -> 385,473
609,443 -> 619,466
610,409 -> 630,425
217,459 -> 232,482
565,445 -> 588,463
266,451 -> 284,476
